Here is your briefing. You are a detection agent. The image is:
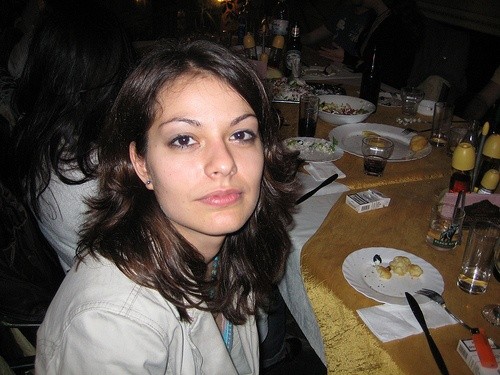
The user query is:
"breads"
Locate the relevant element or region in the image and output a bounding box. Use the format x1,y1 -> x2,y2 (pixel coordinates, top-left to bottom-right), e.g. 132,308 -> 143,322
408,135 -> 428,151
362,131 -> 383,145
376,256 -> 424,279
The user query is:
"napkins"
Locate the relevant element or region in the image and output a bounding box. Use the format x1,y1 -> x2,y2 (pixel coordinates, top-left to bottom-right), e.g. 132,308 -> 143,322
356,303 -> 458,343
303,161 -> 346,182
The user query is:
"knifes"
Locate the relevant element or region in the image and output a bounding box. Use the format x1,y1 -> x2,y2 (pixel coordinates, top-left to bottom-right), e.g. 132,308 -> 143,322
295,174 -> 339,205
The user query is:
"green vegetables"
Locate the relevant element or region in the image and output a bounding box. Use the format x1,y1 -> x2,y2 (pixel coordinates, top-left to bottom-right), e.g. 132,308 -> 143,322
311,83 -> 331,95
287,136 -> 338,152
357,108 -> 368,114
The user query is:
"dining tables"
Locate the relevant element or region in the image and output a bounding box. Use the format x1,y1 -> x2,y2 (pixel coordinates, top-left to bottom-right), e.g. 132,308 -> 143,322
264,43 -> 500,375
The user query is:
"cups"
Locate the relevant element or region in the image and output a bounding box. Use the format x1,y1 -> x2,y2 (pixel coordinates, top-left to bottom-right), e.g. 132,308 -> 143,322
362,135 -> 394,176
425,203 -> 467,250
457,220 -> 500,296
399,85 -> 456,149
405,292 -> 450,375
298,95 -> 320,137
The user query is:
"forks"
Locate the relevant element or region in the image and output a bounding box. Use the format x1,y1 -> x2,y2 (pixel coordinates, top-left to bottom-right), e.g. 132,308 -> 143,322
400,127 -> 431,137
414,288 -> 472,331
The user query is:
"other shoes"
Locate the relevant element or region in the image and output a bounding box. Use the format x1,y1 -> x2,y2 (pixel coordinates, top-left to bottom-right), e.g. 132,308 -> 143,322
261,337 -> 302,372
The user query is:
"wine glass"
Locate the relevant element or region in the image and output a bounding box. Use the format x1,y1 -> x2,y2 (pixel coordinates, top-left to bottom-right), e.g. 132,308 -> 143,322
481,237 -> 500,327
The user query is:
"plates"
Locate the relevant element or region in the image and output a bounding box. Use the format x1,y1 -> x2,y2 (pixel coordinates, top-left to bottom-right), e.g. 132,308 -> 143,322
281,92 -> 433,163
342,247 -> 444,304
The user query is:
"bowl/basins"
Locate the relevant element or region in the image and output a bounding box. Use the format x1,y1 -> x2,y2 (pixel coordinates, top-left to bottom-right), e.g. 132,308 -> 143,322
417,100 -> 442,116
317,95 -> 376,126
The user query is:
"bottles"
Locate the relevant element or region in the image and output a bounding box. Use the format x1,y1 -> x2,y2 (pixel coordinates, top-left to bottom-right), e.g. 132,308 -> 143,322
450,133 -> 500,191
243,0 -> 305,77
360,47 -> 381,113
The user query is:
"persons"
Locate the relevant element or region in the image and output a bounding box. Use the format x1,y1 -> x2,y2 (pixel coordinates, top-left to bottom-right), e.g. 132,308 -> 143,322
0,0 -> 309,375
319,0 -> 427,91
462,63 -> 500,134
301,0 -> 377,56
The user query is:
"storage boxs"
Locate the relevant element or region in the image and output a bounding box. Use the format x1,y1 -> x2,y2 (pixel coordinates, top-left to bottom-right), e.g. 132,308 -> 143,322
345,188 -> 391,213
456,338 -> 500,375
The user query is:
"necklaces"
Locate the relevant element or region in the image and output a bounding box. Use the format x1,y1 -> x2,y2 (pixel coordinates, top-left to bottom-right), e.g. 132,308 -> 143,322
207,254 -> 219,305
